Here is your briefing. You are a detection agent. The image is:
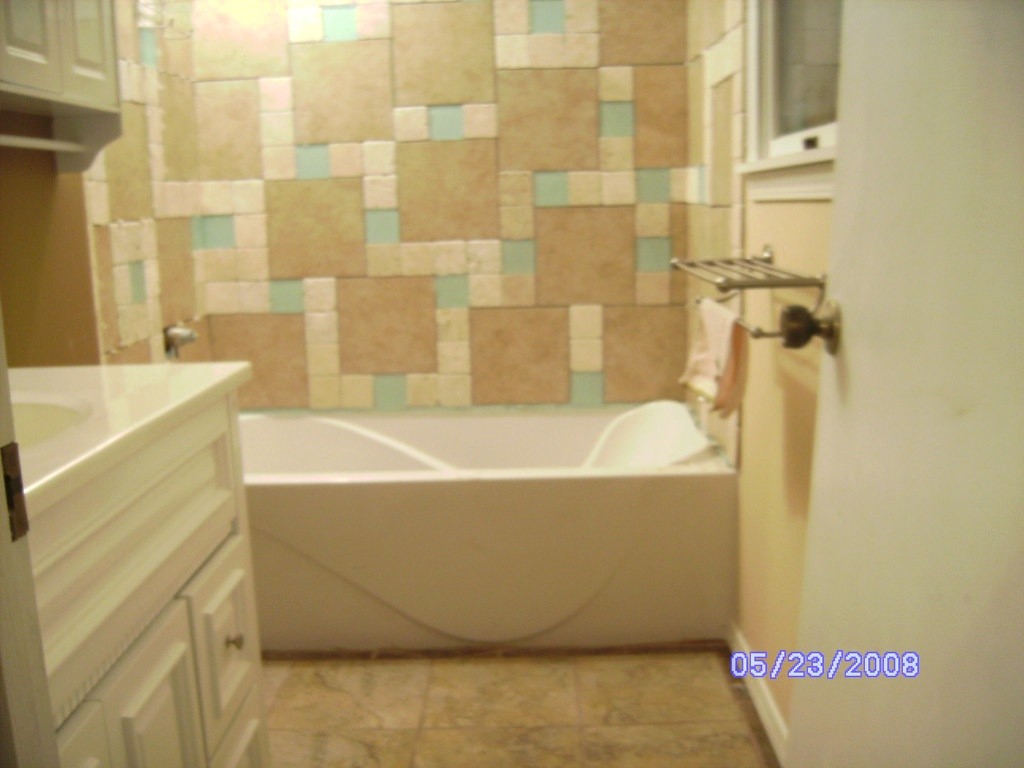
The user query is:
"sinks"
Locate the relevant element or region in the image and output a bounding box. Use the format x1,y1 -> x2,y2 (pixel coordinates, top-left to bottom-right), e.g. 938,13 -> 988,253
12,403 -> 92,448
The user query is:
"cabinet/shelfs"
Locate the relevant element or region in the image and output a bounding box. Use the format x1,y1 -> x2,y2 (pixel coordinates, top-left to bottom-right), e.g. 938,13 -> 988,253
0,0 -> 124,168
24,384 -> 268,768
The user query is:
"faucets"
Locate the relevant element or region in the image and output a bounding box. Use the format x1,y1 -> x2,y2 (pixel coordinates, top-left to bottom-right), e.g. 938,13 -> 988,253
677,357 -> 695,385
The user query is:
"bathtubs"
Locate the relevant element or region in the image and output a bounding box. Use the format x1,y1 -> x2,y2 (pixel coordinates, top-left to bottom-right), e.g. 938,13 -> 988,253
239,400 -> 737,653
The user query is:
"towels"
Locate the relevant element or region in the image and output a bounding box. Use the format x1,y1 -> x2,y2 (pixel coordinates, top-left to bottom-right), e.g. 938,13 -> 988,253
689,297 -> 739,400
